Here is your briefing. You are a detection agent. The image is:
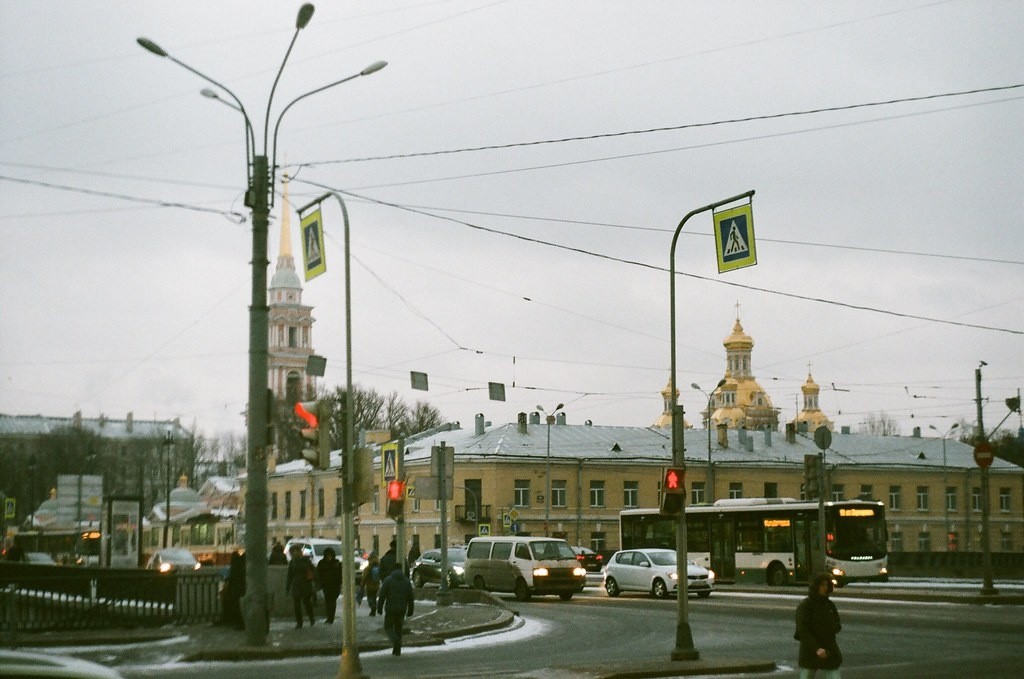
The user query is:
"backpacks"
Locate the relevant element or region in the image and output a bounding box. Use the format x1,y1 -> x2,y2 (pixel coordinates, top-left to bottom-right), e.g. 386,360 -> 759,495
371,566 -> 380,583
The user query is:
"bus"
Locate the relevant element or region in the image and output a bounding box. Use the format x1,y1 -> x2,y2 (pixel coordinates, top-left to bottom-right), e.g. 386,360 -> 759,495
12,530 -> 101,568
618,496 -> 890,588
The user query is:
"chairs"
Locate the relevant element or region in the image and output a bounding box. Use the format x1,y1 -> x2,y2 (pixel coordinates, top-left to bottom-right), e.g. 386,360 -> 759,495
517,547 -> 526,557
620,559 -> 630,564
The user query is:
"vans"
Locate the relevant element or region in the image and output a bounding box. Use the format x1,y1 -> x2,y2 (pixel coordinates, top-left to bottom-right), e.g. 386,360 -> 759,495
464,535 -> 587,604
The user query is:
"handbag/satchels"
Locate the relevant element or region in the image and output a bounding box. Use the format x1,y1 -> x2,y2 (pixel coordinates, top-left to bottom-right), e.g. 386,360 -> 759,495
307,569 -> 314,580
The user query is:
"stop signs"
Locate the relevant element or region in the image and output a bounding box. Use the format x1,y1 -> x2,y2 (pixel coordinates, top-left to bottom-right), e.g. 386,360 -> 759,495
973,442 -> 995,468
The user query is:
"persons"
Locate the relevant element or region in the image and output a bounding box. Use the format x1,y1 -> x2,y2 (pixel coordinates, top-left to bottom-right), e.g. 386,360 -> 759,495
377,563 -> 415,656
221,550 -> 246,629
794,571 -> 843,679
409,545 -> 420,564
357,551 -> 380,617
316,548 -> 342,624
380,540 -> 410,582
286,544 -> 316,630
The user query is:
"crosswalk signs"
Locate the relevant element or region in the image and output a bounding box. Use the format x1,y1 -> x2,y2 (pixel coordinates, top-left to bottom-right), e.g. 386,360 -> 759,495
384,444 -> 399,479
712,204 -> 757,273
298,208 -> 326,279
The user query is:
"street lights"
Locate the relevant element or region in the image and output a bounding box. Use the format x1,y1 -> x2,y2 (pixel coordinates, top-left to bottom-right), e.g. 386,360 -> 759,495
691,382 -> 711,466
132,2 -> 390,643
537,402 -> 565,537
161,429 -> 177,523
929,423 -> 960,551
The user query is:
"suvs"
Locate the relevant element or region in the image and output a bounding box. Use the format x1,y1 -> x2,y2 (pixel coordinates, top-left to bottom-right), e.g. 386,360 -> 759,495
283,538 -> 370,583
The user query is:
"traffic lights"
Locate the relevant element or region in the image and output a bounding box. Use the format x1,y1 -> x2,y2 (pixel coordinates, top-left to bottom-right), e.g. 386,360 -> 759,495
387,480 -> 402,518
662,467 -> 685,513
293,398 -> 332,470
802,453 -> 820,499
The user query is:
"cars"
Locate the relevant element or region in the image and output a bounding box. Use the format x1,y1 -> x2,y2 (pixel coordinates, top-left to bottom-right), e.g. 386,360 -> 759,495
144,548 -> 201,572
410,546 -> 467,592
601,547 -> 716,600
559,546 -> 603,571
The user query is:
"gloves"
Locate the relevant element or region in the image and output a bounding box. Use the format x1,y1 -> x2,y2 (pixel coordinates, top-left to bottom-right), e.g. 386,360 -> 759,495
408,610 -> 413,616
378,609 -> 382,615
